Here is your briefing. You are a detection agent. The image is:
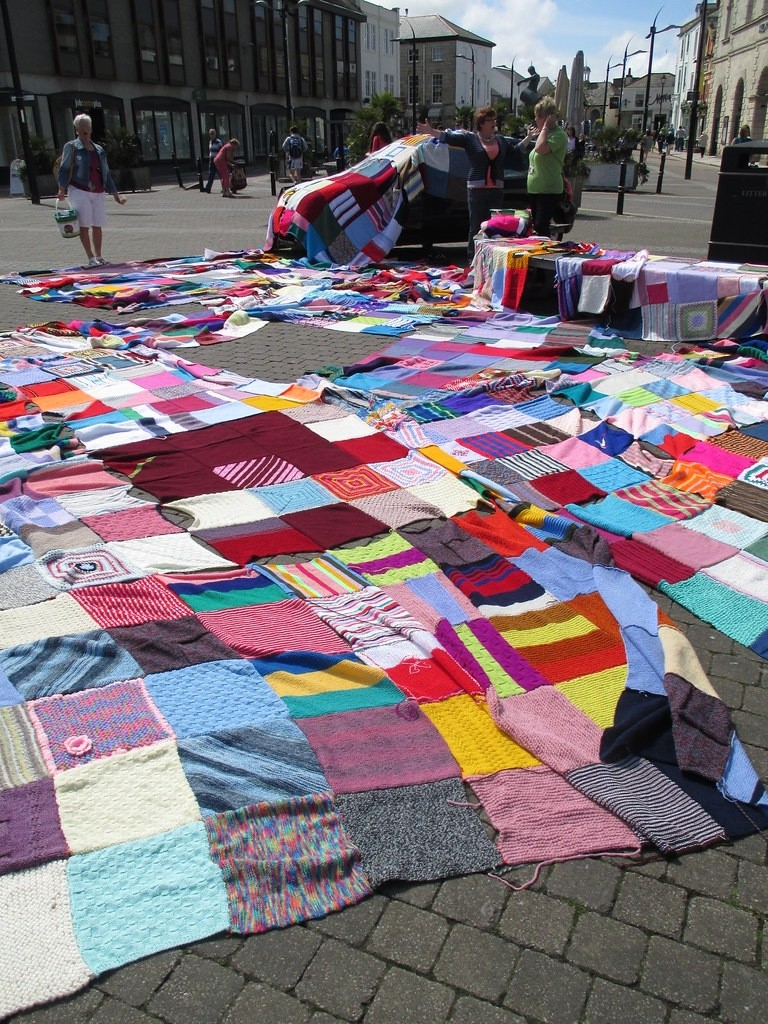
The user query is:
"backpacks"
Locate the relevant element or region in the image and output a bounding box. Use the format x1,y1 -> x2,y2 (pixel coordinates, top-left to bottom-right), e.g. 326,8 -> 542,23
289,135 -> 302,159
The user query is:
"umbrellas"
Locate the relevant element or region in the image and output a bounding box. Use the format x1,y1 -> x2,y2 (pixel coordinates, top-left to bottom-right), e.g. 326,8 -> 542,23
568,51 -> 585,138
554,65 -> 570,126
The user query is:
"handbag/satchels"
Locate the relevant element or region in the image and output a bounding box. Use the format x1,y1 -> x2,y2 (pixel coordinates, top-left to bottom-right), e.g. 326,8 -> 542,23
229,164 -> 248,190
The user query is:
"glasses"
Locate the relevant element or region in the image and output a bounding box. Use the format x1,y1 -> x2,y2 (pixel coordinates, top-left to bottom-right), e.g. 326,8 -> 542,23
483,119 -> 497,123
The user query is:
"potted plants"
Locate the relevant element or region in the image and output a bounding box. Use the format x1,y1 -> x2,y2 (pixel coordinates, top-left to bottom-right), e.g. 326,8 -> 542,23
14,127 -> 152,200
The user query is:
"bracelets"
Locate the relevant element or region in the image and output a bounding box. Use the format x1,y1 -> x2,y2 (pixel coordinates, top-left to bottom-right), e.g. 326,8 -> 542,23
542,127 -> 549,131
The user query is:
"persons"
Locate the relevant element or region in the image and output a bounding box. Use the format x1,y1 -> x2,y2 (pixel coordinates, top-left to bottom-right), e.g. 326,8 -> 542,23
699,131 -> 708,158
730,125 -> 752,145
365,121 -> 392,156
622,123 -> 686,164
416,106 -> 541,265
56,114 -> 127,265
281,125 -> 309,185
202,128 -> 223,193
437,122 -> 464,132
527,95 -> 568,288
334,143 -> 348,157
212,138 -> 241,197
517,66 -> 541,91
511,123 -> 586,169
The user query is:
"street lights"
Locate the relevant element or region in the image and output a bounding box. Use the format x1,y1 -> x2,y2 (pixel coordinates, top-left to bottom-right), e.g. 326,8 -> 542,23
602,63 -> 623,125
617,50 -> 649,129
453,55 -> 474,107
254,0 -> 293,133
493,65 -> 513,110
390,38 -> 416,135
640,24 -> 682,163
656,75 -> 666,131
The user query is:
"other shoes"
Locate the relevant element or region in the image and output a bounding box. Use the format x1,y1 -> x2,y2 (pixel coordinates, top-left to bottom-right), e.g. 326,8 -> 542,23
89,256 -> 105,266
221,191 -> 233,197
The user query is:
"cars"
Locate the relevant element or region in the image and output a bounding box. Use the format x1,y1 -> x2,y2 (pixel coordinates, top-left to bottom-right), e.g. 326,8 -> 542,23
276,137 -> 577,258
